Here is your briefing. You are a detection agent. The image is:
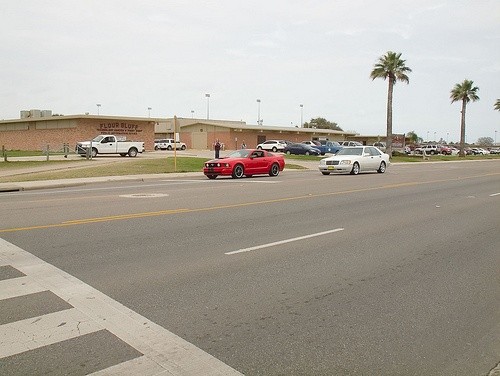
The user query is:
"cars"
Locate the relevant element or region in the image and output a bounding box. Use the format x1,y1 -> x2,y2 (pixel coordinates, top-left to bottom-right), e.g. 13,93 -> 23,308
203,149 -> 285,180
490,148 -> 500,154
282,141 -> 393,155
317,146 -> 389,176
437,145 -> 485,155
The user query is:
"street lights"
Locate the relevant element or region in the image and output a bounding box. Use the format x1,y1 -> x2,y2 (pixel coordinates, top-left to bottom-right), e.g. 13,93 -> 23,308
256,99 -> 261,126
96,103 -> 102,116
494,130 -> 498,145
299,104 -> 303,128
148,107 -> 152,117
205,94 -> 210,120
191,110 -> 194,118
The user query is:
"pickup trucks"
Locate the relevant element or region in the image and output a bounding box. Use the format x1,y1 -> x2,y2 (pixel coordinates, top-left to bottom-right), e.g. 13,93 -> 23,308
75,134 -> 145,158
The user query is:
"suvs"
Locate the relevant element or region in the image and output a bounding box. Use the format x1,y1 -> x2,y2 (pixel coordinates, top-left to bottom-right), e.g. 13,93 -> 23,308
255,140 -> 287,152
154,138 -> 187,151
415,145 -> 438,155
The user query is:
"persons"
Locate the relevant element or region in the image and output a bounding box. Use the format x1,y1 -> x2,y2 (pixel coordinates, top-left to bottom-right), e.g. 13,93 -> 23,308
241,140 -> 247,156
254,153 -> 258,156
215,142 -> 221,158
